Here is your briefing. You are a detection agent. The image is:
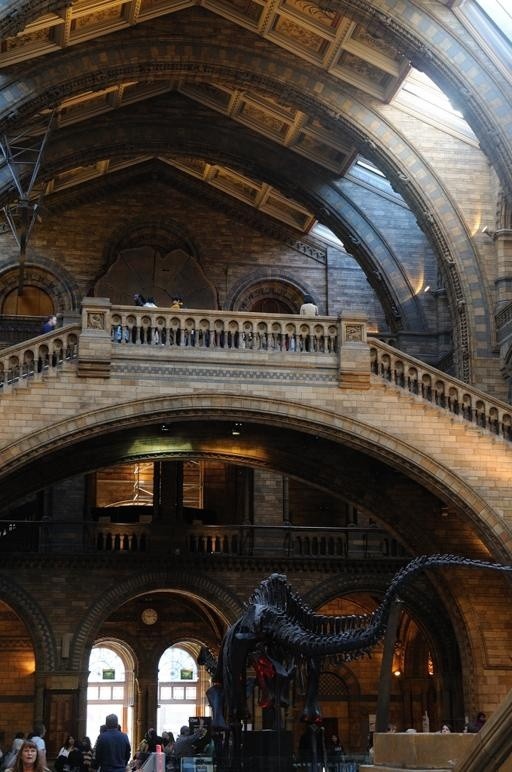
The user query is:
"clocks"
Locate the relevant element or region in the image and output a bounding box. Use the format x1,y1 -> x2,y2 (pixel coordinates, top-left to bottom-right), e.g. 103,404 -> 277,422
141,606 -> 160,626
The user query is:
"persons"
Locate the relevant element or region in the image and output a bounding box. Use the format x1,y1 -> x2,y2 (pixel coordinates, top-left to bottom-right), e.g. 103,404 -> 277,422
441,724 -> 453,733
1,722 -> 50,772
174,717 -> 234,771
53,736 -> 95,772
91,714 -> 131,772
130,728 -> 175,771
300,294 -> 320,352
36,315 -> 58,373
114,290 -> 185,344
329,733 -> 344,755
472,712 -> 487,733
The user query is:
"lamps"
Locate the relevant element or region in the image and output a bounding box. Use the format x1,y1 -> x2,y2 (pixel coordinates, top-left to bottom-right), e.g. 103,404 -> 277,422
478,223 -> 496,241
392,639 -> 435,678
421,283 -> 437,299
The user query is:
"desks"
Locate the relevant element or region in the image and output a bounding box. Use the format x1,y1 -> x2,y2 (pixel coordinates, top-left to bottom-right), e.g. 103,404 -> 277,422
359,731 -> 478,771
